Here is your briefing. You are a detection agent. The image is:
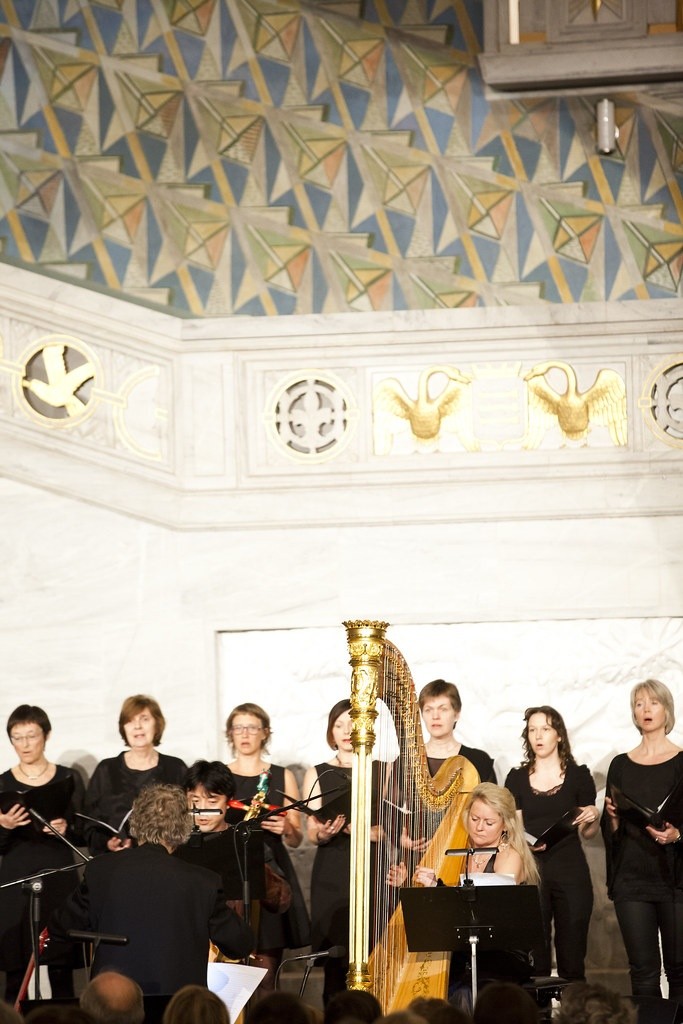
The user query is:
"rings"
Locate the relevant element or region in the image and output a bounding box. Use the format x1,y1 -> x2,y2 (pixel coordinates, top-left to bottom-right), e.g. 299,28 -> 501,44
653,837 -> 661,842
329,826 -> 336,830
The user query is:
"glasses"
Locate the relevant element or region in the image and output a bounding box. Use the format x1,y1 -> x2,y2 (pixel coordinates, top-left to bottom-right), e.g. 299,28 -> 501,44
232,725 -> 261,736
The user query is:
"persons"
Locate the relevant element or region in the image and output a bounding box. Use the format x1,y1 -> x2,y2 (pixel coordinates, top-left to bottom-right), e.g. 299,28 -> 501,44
173,760 -> 294,967
0,676 -> 683,1024
384,781 -> 543,892
55,786 -> 254,1023
0,677 -> 683,1024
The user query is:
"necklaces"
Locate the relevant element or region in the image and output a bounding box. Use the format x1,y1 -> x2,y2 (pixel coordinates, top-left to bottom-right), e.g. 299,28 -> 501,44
18,761 -> 49,780
471,852 -> 495,868
333,752 -> 347,767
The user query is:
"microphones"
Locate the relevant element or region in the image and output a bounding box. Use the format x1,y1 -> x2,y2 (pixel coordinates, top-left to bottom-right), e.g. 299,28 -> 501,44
287,946 -> 347,963
332,769 -> 352,782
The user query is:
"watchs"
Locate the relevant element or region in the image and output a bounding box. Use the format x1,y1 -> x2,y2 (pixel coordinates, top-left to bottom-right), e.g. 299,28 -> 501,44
673,833 -> 683,844
